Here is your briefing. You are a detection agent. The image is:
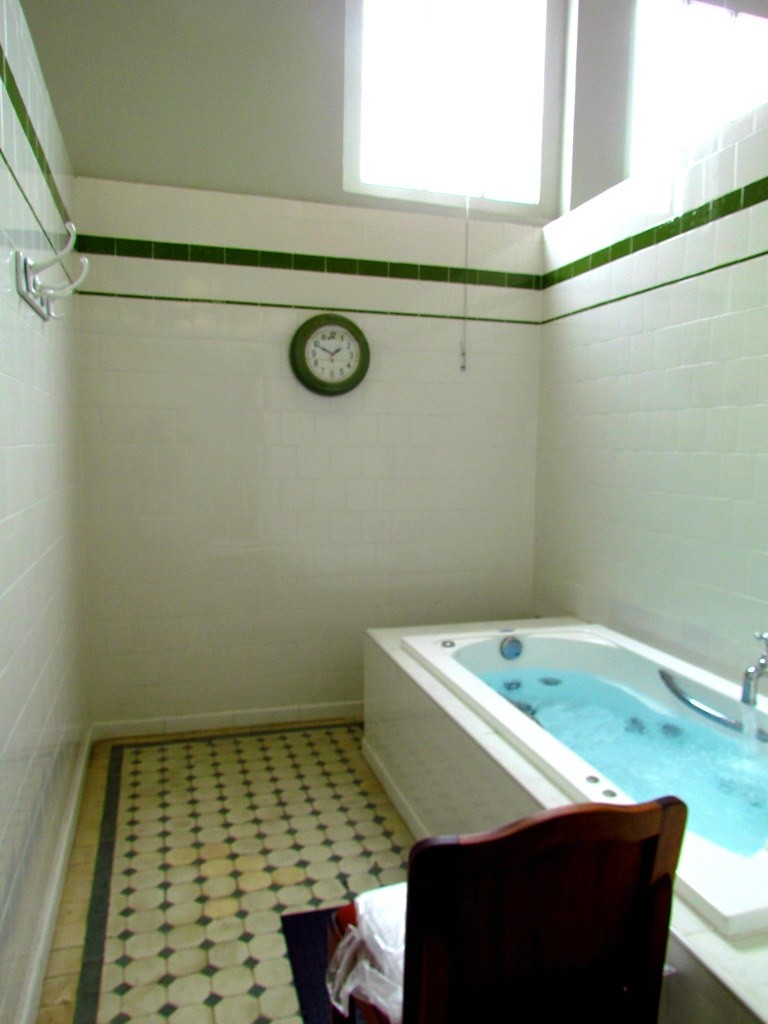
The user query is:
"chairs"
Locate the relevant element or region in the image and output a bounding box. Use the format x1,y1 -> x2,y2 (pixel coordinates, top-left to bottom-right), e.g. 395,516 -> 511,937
324,794 -> 687,1024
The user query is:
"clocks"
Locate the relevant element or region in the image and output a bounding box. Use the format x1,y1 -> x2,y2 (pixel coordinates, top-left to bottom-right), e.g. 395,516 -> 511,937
288,313 -> 370,396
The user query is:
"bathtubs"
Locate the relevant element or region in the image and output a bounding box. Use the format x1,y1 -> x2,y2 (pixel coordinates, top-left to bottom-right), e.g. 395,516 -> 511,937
360,614 -> 767,1024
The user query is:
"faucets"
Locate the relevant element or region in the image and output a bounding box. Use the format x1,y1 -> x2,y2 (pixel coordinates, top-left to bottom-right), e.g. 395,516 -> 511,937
740,631 -> 768,707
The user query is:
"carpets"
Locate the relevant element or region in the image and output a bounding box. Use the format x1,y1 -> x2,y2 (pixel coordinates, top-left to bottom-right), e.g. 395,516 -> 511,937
281,904 -> 345,1024
